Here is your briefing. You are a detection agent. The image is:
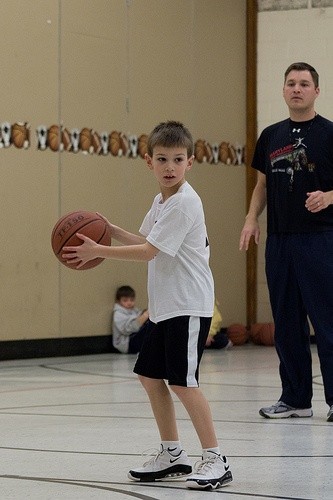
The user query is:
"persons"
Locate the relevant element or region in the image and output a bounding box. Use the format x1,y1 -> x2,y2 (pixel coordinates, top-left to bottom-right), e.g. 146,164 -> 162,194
61,119 -> 233,490
113,285 -> 150,354
206,300 -> 231,349
239,63 -> 333,421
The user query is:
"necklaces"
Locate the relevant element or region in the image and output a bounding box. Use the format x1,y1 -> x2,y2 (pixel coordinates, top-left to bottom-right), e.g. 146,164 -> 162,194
289,112 -> 316,191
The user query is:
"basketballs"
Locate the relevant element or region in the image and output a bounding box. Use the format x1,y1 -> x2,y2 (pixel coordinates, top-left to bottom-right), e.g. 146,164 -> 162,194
251,322 -> 274,346
51,210 -> 113,271
228,323 -> 250,345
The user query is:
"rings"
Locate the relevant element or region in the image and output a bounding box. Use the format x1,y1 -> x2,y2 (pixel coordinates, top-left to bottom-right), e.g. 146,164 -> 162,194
318,202 -> 321,206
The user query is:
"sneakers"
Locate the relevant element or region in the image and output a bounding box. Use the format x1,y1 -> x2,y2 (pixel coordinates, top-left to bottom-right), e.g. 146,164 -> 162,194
128,448 -> 192,482
326,405 -> 333,422
258,401 -> 313,419
185,450 -> 234,490
